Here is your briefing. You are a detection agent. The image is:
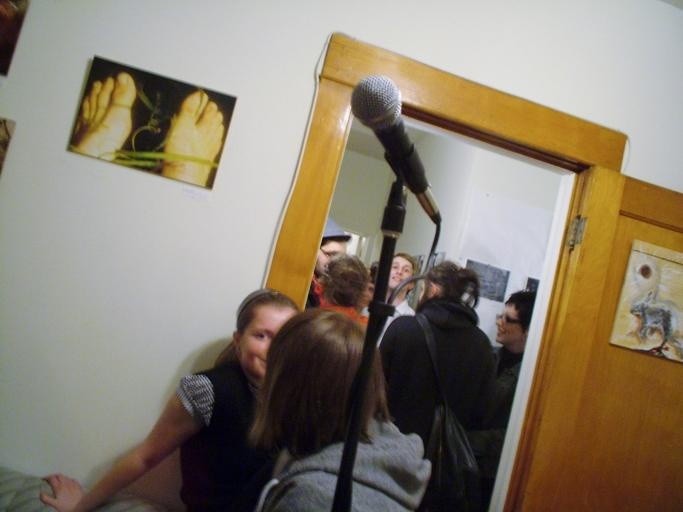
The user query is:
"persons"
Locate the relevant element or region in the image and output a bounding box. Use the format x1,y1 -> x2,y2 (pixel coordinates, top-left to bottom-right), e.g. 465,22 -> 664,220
70,72 -> 226,189
38,212 -> 536,512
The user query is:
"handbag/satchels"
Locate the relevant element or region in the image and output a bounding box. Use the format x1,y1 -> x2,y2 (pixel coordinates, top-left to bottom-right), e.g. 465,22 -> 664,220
425,404 -> 487,512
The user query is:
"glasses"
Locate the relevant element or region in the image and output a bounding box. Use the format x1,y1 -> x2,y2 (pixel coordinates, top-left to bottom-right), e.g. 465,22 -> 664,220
496,314 -> 521,324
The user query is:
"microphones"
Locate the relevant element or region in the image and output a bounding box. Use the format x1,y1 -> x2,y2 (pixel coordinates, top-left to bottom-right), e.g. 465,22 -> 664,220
351,74 -> 441,225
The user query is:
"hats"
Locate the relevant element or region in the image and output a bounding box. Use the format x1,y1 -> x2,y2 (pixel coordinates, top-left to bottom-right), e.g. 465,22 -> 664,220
323,218 -> 351,240
